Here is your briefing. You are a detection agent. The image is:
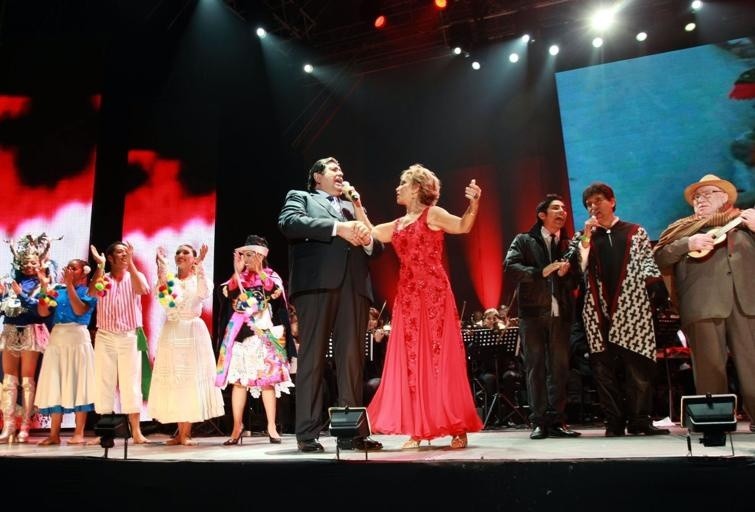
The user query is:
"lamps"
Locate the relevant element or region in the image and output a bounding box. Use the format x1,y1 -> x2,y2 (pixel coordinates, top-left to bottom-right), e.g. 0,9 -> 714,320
95,411 -> 133,460
328,406 -> 372,461
680,394 -> 738,456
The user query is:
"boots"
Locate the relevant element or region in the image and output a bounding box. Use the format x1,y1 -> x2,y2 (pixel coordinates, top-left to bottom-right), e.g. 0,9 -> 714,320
16,376 -> 36,443
0,373 -> 19,443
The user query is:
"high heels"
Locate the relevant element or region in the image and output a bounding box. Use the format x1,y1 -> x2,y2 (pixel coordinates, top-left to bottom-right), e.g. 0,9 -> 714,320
266,427 -> 282,444
450,432 -> 468,449
401,434 -> 432,450
224,423 -> 246,446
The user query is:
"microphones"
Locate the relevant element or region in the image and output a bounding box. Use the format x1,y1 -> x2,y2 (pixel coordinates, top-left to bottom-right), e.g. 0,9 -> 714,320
342,181 -> 359,200
591,212 -> 598,232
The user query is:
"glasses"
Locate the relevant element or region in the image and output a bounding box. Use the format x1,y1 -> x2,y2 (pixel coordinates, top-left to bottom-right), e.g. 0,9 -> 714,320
695,190 -> 724,200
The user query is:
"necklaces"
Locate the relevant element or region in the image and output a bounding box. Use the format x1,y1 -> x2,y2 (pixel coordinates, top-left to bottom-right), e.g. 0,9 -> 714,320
400,203 -> 423,225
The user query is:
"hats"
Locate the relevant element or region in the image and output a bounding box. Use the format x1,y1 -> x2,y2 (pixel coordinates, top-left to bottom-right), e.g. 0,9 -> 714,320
683,174 -> 739,209
235,233 -> 270,258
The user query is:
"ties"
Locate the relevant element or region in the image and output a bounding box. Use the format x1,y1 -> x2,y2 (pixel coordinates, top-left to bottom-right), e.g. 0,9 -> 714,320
328,194 -> 341,214
551,233 -> 560,301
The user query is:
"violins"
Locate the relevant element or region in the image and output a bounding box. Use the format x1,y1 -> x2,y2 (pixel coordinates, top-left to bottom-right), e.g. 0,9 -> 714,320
367,328 -> 390,339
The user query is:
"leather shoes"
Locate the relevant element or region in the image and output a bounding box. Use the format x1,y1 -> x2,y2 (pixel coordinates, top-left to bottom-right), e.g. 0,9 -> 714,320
546,424 -> 581,437
606,423 -> 626,437
627,420 -> 670,435
530,422 -> 554,438
297,438 -> 324,454
336,435 -> 383,450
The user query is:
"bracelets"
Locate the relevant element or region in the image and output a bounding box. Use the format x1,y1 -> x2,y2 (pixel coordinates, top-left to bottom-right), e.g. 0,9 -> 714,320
467,206 -> 477,216
468,204 -> 478,213
580,234 -> 591,243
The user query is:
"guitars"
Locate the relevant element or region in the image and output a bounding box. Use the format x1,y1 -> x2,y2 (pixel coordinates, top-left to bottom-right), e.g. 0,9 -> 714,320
687,217 -> 744,261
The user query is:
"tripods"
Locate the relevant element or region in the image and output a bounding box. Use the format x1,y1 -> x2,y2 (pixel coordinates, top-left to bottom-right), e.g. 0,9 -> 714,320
483,360 -> 532,430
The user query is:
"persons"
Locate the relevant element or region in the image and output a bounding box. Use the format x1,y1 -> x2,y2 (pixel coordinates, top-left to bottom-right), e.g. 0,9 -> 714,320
214,232 -> 298,445
500,192 -> 584,439
153,242 -> 226,447
340,162 -> 483,453
288,304 -> 299,335
30,259 -> 96,447
86,240 -> 153,447
0,232 -> 52,446
367,304 -> 388,398
275,156 -> 384,454
654,173 -> 755,435
578,182 -> 671,439
467,302 -> 522,429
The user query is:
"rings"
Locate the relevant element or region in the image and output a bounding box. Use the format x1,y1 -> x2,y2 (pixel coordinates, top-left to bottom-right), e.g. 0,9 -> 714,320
473,192 -> 479,200
